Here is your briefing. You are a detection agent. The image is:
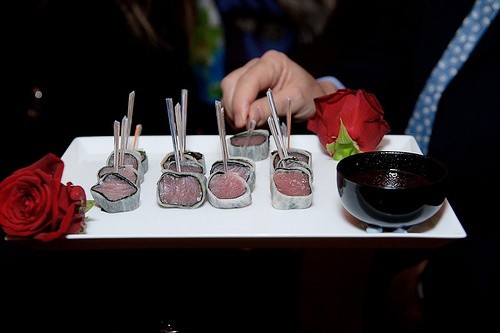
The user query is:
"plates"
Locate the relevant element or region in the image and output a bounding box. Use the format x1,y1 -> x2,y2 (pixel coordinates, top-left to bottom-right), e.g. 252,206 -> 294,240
58,135 -> 467,240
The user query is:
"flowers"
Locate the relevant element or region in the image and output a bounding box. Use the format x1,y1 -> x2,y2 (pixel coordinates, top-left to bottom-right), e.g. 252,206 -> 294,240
307,87 -> 391,161
0,154 -> 97,242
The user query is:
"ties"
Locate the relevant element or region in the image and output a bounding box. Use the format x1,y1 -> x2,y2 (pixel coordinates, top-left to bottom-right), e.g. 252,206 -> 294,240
405,0 -> 500,157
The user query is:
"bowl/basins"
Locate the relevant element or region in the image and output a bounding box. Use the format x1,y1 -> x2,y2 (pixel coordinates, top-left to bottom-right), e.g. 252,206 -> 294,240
335,150 -> 447,229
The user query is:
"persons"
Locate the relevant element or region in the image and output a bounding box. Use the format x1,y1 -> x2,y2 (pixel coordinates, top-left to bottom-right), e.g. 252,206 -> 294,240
220,0 -> 500,333
